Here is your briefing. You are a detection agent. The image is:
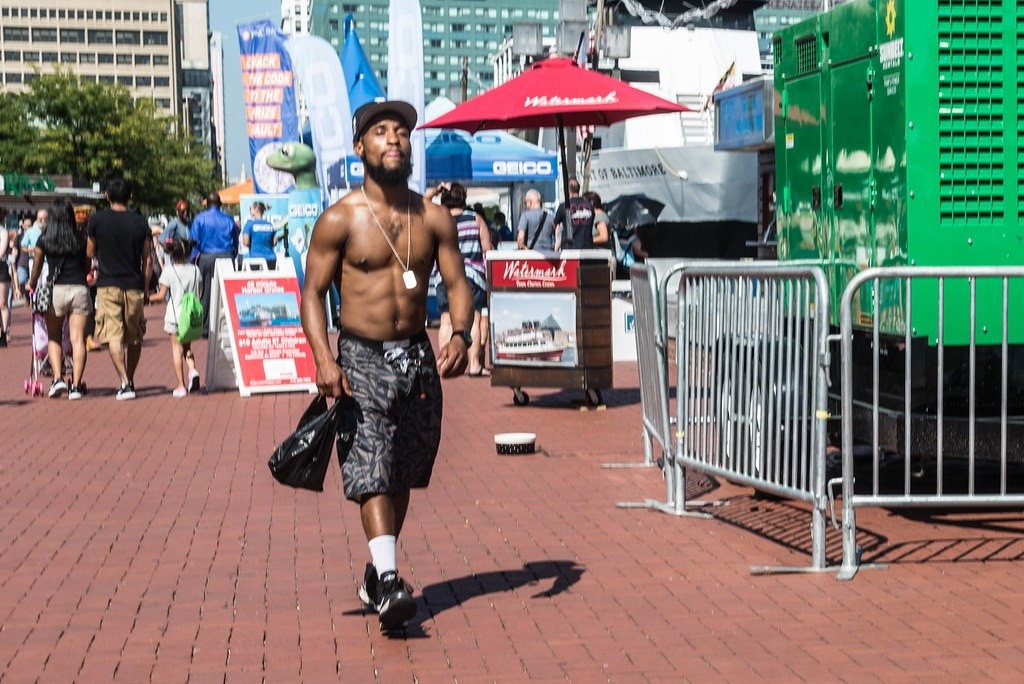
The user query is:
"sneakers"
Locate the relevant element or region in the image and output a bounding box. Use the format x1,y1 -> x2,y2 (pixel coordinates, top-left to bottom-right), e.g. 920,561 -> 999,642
358,561 -> 384,604
68,386 -> 81,400
374,571 -> 418,632
116,384 -> 136,400
188,370 -> 201,392
173,388 -> 186,397
49,378 -> 67,397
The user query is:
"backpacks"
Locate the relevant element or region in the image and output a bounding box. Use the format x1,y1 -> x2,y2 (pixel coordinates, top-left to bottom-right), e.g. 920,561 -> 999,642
168,262 -> 205,344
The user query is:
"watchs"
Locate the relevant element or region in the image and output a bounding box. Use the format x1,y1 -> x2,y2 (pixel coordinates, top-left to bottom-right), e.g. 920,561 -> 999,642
450,329 -> 473,350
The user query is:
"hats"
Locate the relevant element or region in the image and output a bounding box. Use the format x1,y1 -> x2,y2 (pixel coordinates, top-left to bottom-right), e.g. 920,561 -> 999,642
351,101 -> 418,145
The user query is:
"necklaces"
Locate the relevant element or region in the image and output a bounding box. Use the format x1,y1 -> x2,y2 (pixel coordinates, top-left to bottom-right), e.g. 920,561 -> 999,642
361,185 -> 417,289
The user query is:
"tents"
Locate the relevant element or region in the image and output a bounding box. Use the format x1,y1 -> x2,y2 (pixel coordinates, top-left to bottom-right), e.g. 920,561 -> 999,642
325,96 -> 558,214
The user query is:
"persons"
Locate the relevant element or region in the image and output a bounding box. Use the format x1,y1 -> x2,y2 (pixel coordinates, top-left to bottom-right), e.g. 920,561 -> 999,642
300,101 -> 475,631
0,176 -> 278,400
423,181 -> 649,378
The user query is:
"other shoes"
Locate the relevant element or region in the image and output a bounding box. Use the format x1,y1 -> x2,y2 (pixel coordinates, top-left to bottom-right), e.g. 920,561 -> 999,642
0,337 -> 7,347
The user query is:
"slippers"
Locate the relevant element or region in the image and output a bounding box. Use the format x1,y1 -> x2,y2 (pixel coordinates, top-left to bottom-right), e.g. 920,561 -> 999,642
467,366 -> 489,377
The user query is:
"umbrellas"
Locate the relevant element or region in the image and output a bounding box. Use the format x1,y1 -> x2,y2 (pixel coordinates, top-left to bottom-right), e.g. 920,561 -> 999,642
416,54 -> 700,248
603,194 -> 665,230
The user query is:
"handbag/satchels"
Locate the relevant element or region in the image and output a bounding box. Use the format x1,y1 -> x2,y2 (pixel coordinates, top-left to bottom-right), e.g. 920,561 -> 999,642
267,393 -> 342,492
35,285 -> 51,313
